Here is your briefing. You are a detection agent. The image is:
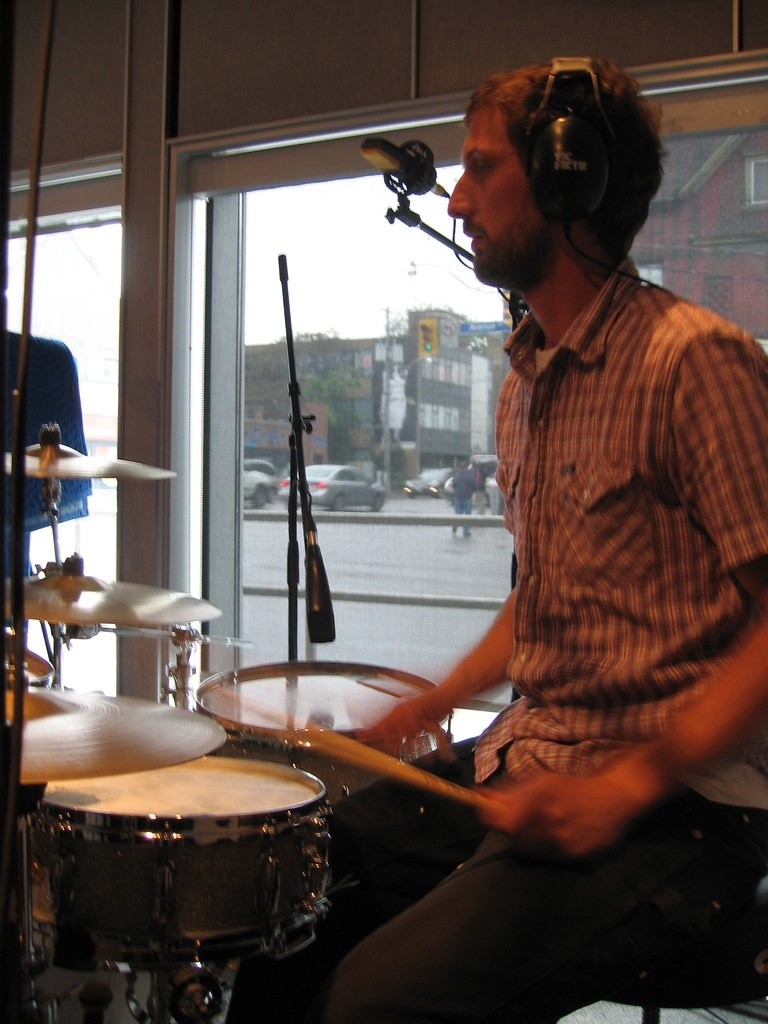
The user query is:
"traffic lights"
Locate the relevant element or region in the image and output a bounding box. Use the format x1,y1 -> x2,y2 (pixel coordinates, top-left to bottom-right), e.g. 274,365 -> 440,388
418,318 -> 440,358
501,294 -> 513,331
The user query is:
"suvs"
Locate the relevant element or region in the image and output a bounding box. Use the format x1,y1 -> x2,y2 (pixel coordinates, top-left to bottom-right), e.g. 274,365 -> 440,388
243,459 -> 279,506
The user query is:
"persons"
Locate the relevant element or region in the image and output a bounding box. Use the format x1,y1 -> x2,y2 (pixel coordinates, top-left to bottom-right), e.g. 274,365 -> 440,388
444,458 -> 489,536
223,55 -> 768,1024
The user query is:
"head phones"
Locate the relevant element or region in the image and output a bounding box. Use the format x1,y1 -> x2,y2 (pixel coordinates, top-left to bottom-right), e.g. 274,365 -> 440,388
525,56 -> 617,222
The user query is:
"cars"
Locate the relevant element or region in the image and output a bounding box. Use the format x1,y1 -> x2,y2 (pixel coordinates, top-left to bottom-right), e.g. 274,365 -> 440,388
403,468 -> 453,495
277,464 -> 389,510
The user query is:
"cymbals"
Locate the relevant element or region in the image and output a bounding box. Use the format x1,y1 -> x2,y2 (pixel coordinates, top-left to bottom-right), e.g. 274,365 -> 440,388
4,578 -> 225,629
4,685 -> 227,786
0,443 -> 183,482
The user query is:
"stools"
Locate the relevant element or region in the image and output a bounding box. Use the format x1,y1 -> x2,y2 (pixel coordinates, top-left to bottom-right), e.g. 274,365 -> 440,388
594,875 -> 768,1024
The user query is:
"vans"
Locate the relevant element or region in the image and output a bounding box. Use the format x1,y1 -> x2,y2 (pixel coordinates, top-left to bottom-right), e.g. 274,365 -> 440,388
444,454 -> 498,495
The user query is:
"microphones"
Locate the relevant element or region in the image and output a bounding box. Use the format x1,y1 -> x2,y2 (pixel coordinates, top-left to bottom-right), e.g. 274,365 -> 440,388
304,518 -> 336,644
361,138 -> 450,199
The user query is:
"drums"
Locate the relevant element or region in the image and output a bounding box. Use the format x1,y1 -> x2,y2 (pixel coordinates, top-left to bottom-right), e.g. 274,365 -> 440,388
203,659 -> 447,799
34,757 -> 327,963
